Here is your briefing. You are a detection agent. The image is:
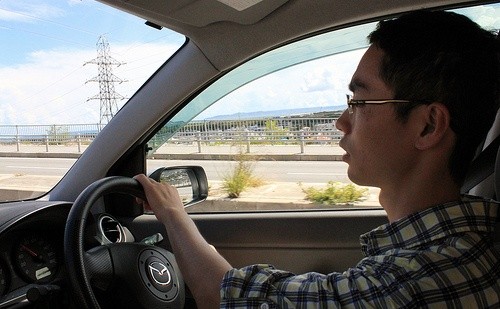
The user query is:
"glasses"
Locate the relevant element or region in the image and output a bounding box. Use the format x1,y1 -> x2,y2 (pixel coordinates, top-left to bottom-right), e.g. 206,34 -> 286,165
347,94 -> 409,114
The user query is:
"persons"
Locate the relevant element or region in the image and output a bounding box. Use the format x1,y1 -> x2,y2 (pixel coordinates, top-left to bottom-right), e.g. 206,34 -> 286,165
133,9 -> 500,308
